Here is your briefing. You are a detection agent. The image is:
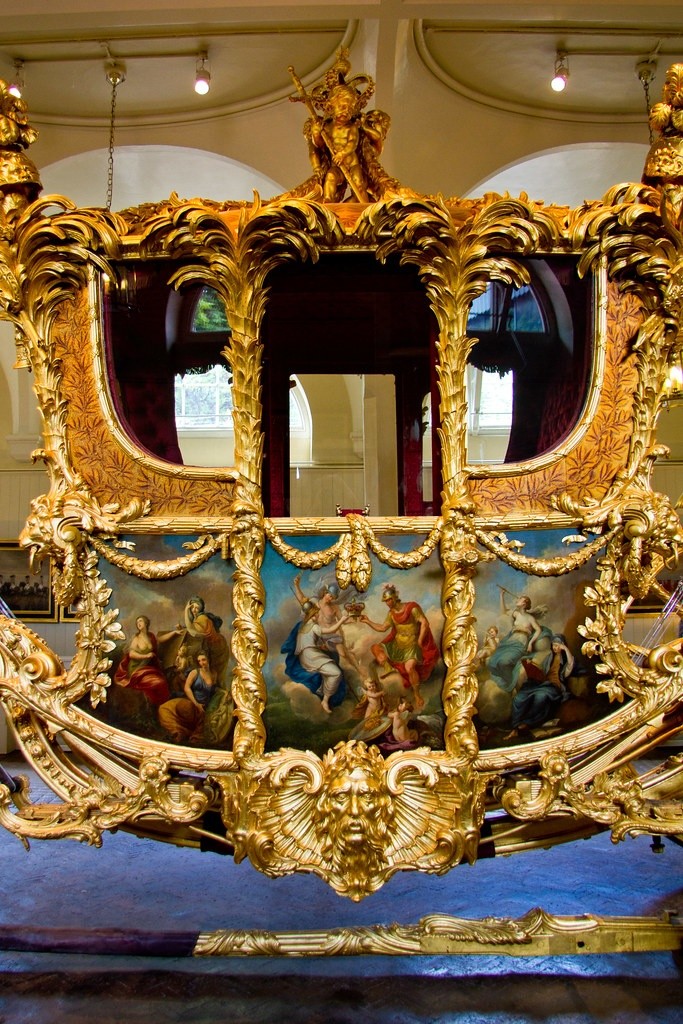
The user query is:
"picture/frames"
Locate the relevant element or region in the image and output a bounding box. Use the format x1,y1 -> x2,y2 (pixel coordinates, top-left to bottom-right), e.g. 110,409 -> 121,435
59,604 -> 81,624
0,539 -> 59,623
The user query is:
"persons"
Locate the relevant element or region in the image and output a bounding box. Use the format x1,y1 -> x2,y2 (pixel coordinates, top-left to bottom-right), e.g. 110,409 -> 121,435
1,80 -> 41,194
1,575 -> 48,609
643,64 -> 683,185
308,86 -> 369,202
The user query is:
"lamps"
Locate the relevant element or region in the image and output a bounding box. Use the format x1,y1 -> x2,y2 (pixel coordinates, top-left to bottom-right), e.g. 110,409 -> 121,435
194,51 -> 212,96
551,49 -> 571,92
8,57 -> 26,98
